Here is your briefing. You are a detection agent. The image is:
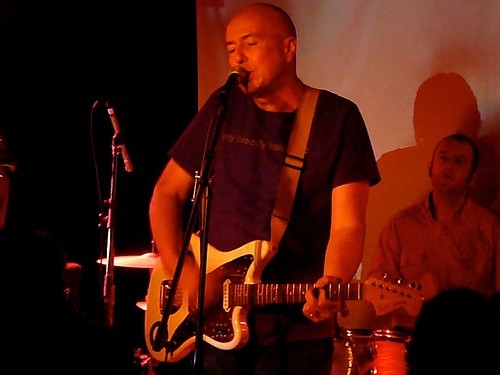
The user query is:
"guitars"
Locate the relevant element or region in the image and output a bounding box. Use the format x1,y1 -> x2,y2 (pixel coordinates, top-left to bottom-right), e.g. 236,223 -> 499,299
143,232 -> 424,362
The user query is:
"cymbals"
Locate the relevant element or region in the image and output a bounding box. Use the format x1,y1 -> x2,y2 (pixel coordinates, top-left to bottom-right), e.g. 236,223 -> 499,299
96,239 -> 159,270
135,300 -> 147,311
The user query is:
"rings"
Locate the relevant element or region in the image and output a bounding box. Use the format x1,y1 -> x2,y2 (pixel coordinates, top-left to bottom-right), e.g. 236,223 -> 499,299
310,312 -> 317,317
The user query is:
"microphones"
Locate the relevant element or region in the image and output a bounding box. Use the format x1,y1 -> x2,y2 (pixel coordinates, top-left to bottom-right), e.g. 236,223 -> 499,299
107,102 -> 133,172
219,66 -> 247,98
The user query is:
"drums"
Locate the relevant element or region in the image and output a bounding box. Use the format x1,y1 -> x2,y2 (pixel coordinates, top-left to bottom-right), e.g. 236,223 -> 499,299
329,330 -> 411,375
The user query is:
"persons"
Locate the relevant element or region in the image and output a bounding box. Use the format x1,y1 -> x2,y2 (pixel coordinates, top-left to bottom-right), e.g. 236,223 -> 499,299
148,3 -> 381,375
366,133 -> 500,375
